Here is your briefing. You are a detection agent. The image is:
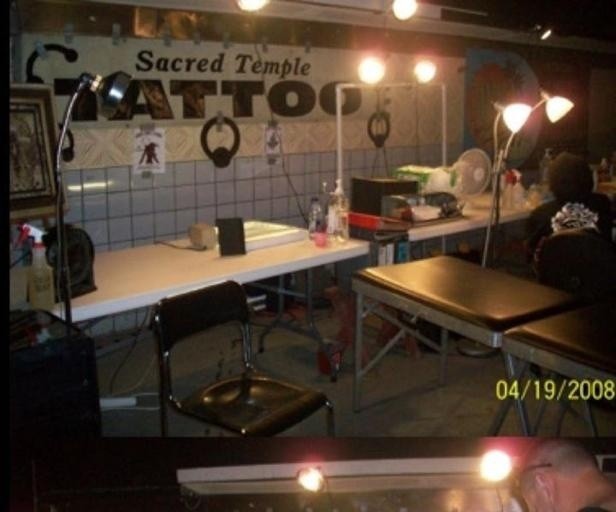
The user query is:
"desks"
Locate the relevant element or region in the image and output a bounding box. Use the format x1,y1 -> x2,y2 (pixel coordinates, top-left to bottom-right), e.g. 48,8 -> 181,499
349,191 -> 555,245
51,220 -> 372,416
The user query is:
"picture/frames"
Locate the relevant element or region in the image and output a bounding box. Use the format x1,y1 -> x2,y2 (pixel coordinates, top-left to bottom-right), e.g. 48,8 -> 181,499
8,80 -> 72,224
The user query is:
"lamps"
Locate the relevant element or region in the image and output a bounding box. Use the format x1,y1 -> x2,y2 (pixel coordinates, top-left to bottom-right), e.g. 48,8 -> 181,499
491,99 -> 535,158
53,67 -> 134,175
502,90 -> 575,158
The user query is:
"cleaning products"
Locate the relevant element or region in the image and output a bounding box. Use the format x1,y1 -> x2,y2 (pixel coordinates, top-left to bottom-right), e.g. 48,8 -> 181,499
12,221 -> 54,313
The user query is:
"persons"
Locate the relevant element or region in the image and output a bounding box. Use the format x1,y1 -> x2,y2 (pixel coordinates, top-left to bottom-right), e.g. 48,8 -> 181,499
517,153 -> 616,287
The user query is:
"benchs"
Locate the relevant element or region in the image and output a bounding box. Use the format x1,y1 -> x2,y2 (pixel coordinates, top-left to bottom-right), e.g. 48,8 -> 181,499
348,254 -> 616,438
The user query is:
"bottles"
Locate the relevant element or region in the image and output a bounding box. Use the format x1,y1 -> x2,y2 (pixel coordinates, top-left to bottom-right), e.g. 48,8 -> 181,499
502,182 -> 512,211
28,244 -> 56,313
309,196 -> 323,239
512,183 -> 524,210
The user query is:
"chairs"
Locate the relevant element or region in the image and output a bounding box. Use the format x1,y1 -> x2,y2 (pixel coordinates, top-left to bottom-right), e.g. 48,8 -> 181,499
534,224 -> 602,261
149,277 -> 339,438
